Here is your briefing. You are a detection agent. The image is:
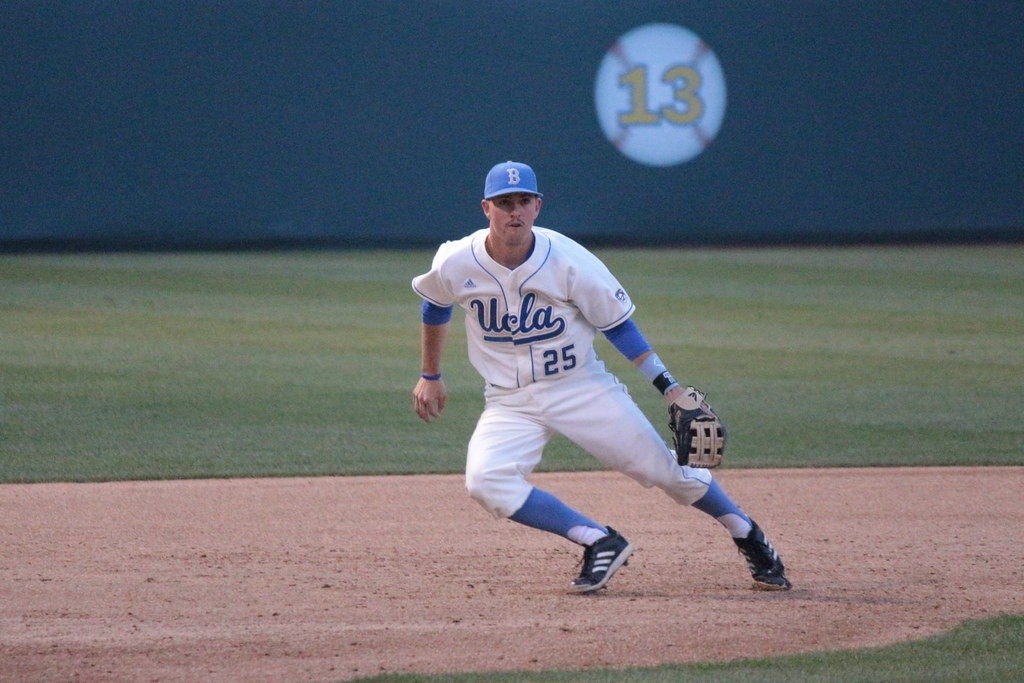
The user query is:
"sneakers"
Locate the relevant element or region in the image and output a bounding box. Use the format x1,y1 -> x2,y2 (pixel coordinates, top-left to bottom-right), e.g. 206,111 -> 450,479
571,526 -> 633,591
732,519 -> 793,590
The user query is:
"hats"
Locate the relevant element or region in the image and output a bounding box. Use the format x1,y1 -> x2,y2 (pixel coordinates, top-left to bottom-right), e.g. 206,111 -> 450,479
484,160 -> 543,200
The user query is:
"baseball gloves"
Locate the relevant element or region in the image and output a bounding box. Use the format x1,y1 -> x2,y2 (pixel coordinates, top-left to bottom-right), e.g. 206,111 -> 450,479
669,385 -> 727,470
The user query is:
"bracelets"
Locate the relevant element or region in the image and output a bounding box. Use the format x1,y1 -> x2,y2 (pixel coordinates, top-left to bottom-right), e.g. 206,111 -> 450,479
423,373 -> 440,380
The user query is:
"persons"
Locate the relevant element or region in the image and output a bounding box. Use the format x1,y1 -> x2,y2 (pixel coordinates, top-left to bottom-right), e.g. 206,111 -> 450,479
412,162 -> 786,592
